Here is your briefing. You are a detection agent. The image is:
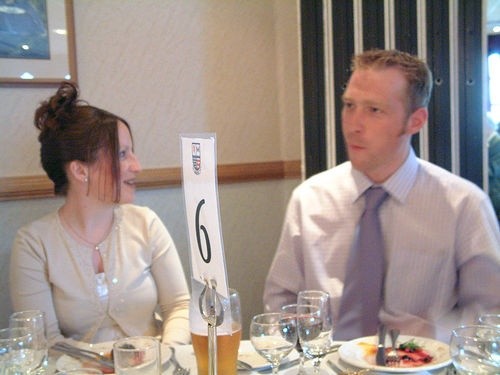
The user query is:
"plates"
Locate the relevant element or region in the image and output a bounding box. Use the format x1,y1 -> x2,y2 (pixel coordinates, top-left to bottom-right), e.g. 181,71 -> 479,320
338,333 -> 456,372
51,337 -> 171,375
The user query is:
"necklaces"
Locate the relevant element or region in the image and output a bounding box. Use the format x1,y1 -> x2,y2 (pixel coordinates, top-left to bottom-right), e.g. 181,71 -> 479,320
59,205 -> 114,251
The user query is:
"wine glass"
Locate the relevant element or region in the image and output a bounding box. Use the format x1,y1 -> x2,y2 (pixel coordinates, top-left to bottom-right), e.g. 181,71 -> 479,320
298,289 -> 334,375
280,303 -> 323,375
248,312 -> 299,375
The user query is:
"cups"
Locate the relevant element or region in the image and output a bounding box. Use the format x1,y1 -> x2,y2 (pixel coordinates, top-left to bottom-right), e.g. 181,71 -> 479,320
112,337 -> 162,375
188,289 -> 241,375
450,313 -> 500,375
0,327 -> 34,375
10,310 -> 49,375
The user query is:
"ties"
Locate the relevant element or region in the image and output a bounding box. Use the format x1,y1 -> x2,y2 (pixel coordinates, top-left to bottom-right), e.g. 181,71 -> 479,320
332,188 -> 388,341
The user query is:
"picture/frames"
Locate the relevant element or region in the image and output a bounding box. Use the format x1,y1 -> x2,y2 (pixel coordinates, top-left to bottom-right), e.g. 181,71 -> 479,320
0,0 -> 78,84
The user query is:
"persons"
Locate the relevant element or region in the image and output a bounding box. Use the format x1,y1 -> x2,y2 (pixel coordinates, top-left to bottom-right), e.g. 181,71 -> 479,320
263,49 -> 500,350
487,121 -> 500,214
9,80 -> 191,374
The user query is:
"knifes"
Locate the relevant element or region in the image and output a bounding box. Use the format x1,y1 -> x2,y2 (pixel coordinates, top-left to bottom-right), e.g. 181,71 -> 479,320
375,324 -> 388,366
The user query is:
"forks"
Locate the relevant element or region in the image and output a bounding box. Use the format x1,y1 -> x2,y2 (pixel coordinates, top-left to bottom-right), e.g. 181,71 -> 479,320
388,327 -> 400,367
